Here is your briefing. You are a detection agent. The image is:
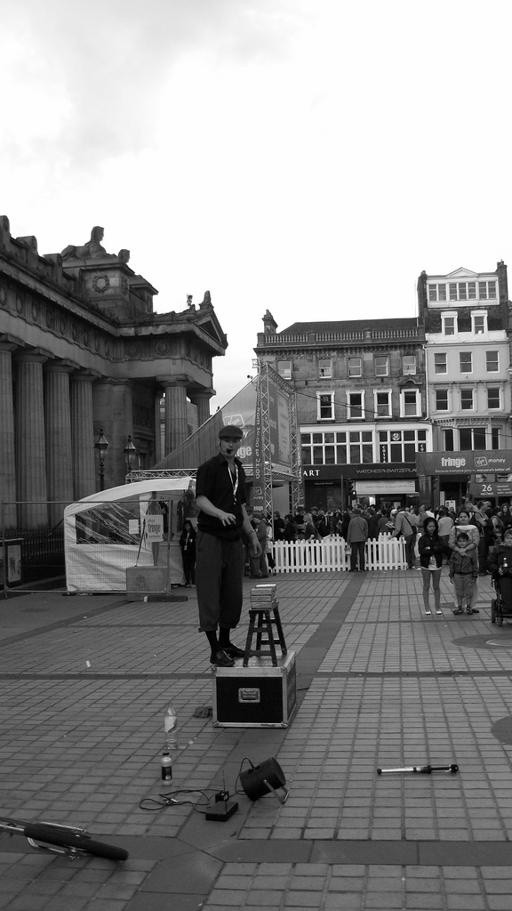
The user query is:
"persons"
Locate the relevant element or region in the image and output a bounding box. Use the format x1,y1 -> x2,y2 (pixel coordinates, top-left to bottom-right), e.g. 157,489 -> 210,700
192,423 -> 263,668
179,520 -> 197,590
244,500 -> 511,616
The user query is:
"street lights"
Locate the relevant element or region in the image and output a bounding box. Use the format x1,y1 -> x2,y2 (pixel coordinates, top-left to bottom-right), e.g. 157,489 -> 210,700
94,428 -> 109,491
123,434 -> 138,486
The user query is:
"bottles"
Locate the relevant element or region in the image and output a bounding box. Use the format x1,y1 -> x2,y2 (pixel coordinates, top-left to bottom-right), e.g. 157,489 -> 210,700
160,752 -> 173,786
164,699 -> 177,749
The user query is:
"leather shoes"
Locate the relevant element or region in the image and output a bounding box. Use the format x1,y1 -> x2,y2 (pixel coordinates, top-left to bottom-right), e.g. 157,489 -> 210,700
210,650 -> 234,666
425,611 -> 431,615
224,645 -> 245,657
436,611 -> 443,615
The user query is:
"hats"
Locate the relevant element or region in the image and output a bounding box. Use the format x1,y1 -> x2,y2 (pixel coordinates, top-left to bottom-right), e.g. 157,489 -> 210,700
219,426 -> 243,438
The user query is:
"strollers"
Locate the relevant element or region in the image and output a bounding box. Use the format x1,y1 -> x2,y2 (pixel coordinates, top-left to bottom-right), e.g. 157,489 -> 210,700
483,529 -> 512,628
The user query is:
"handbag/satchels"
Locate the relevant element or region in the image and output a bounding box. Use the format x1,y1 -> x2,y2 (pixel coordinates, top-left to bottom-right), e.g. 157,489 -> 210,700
412,526 -> 417,535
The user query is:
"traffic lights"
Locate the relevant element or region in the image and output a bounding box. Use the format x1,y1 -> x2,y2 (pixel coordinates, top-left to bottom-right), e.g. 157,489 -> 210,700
352,490 -> 358,499
347,478 -> 354,495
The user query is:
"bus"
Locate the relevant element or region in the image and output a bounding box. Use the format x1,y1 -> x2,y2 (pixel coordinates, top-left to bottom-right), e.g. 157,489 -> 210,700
463,468 -> 512,511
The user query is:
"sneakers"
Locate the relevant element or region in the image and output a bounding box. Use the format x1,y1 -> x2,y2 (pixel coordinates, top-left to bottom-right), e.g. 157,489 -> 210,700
453,607 -> 479,615
